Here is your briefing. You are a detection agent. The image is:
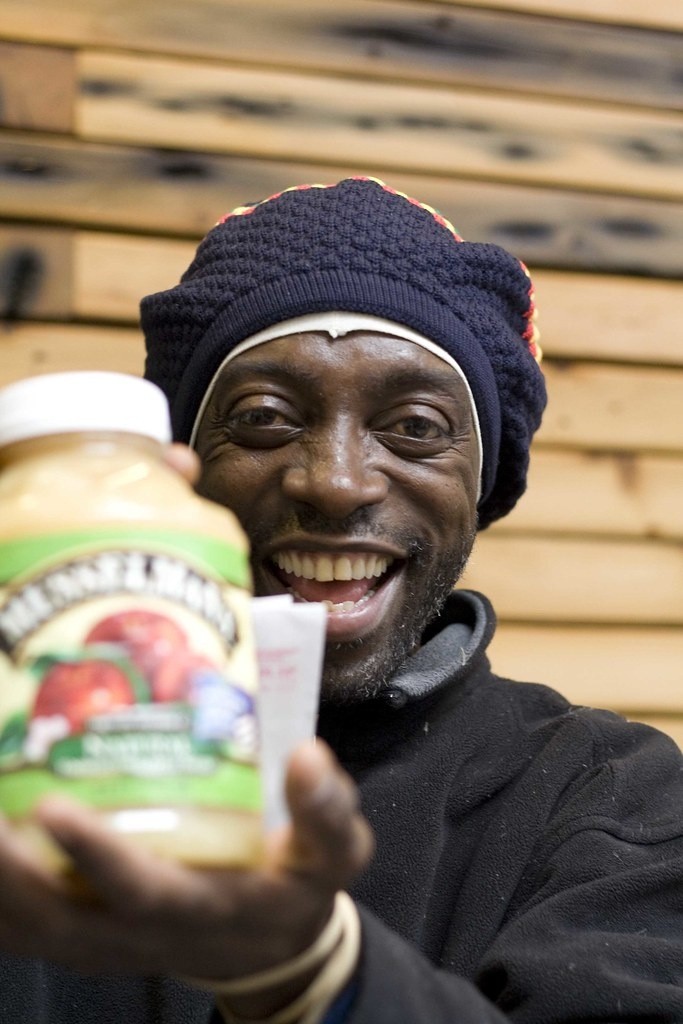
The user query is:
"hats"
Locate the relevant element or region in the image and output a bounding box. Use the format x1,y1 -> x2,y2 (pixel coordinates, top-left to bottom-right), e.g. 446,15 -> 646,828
139,175 -> 548,530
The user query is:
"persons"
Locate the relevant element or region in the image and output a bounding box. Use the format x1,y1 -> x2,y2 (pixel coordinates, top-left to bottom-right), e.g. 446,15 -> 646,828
0,170 -> 683,1023
